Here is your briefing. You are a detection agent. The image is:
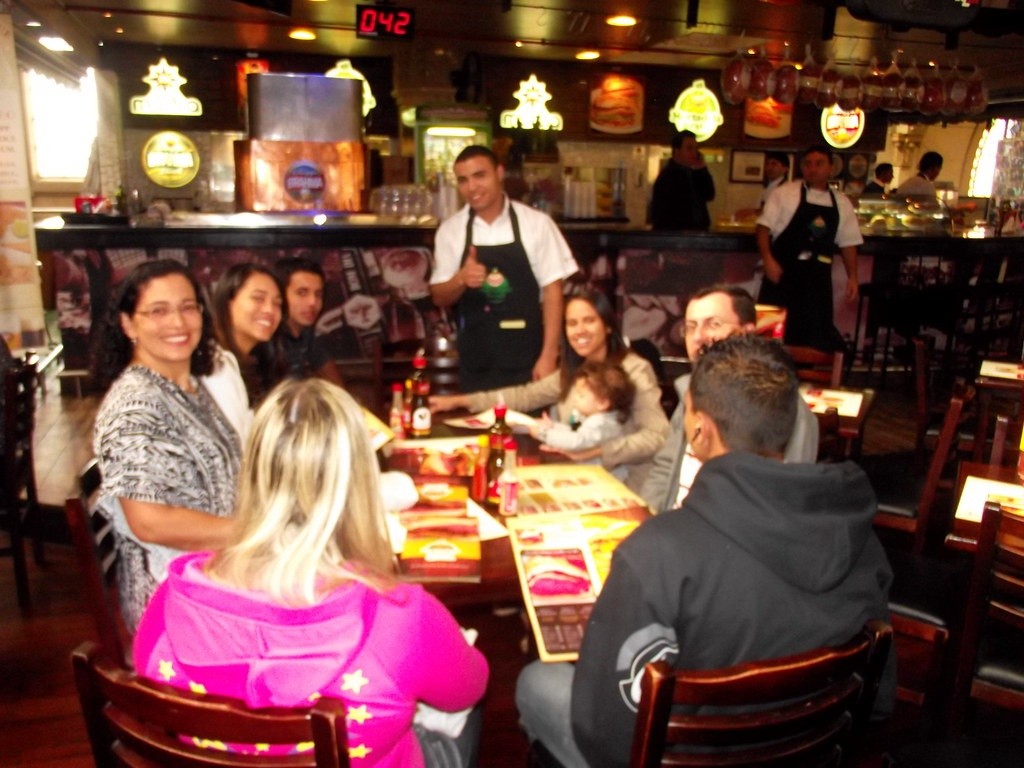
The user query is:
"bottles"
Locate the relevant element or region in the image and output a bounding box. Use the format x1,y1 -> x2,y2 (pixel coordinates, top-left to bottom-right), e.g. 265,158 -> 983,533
390,378 -> 413,439
487,404 -> 512,505
471,434 -> 490,501
405,357 -> 428,434
411,383 -> 432,439
499,441 -> 518,516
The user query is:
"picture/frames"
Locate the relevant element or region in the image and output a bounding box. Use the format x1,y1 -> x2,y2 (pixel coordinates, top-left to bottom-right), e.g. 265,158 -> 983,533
729,149 -> 765,184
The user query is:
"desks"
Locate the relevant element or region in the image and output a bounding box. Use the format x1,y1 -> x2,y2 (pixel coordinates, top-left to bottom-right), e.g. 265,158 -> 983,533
386,459 -> 657,768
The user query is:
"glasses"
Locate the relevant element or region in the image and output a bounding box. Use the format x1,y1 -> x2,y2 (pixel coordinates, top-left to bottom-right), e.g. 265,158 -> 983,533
131,303 -> 203,322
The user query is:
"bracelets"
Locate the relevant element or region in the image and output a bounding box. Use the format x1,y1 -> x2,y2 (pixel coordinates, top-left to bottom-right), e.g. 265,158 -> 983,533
765,261 -> 777,270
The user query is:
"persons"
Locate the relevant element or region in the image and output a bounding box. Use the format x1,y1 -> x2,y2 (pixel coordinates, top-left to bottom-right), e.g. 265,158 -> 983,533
861,163 -> 894,193
270,256 -> 347,390
428,145 -> 579,392
131,376 -> 489,768
515,327 -> 894,768
646,129 -> 716,230
529,360 -> 636,483
427,289 -> 673,495
212,262 -> 289,412
639,284 -> 818,514
37,200 -> 188,347
890,151 -> 943,206
756,142 -> 864,359
84,256 -> 250,638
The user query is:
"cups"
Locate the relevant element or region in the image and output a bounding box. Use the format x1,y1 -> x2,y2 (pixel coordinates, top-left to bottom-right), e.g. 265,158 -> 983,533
370,185 -> 434,219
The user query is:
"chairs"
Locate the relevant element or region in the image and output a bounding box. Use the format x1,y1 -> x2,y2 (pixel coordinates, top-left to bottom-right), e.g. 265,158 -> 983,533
0,244 -> 1024,768
